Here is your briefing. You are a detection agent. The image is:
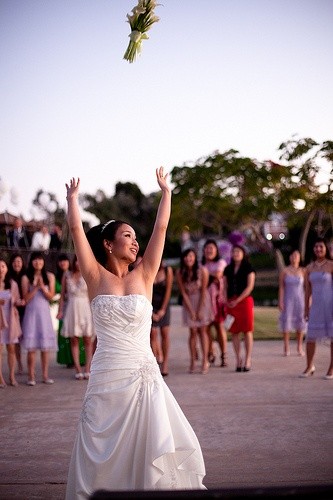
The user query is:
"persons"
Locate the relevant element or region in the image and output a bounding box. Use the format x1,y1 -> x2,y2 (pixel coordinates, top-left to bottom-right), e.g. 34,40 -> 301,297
299,240 -> 333,380
4,216 -> 28,252
21,251 -> 55,387
63,166 -> 203,499
27,224 -> 49,259
46,221 -> 67,258
217,244 -> 255,373
56,251 -> 88,369
199,237 -> 229,370
272,249 -> 306,358
173,247 -> 212,376
145,253 -> 171,379
66,253 -> 96,382
7,253 -> 31,376
0,259 -> 24,389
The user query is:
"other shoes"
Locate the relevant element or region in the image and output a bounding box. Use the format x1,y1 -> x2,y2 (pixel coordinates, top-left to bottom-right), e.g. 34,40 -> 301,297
0,368 -> 90,389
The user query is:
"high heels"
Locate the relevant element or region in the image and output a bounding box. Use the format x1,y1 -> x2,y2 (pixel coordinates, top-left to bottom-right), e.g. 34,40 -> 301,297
299,365 -> 316,378
154,348 -> 303,377
324,373 -> 333,379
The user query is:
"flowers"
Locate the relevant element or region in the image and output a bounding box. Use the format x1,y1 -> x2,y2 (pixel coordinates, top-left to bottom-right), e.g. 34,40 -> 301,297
122,0 -> 163,64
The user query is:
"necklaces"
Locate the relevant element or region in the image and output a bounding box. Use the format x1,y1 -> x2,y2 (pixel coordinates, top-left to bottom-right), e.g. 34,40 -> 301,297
315,259 -> 327,267
289,266 -> 300,273
